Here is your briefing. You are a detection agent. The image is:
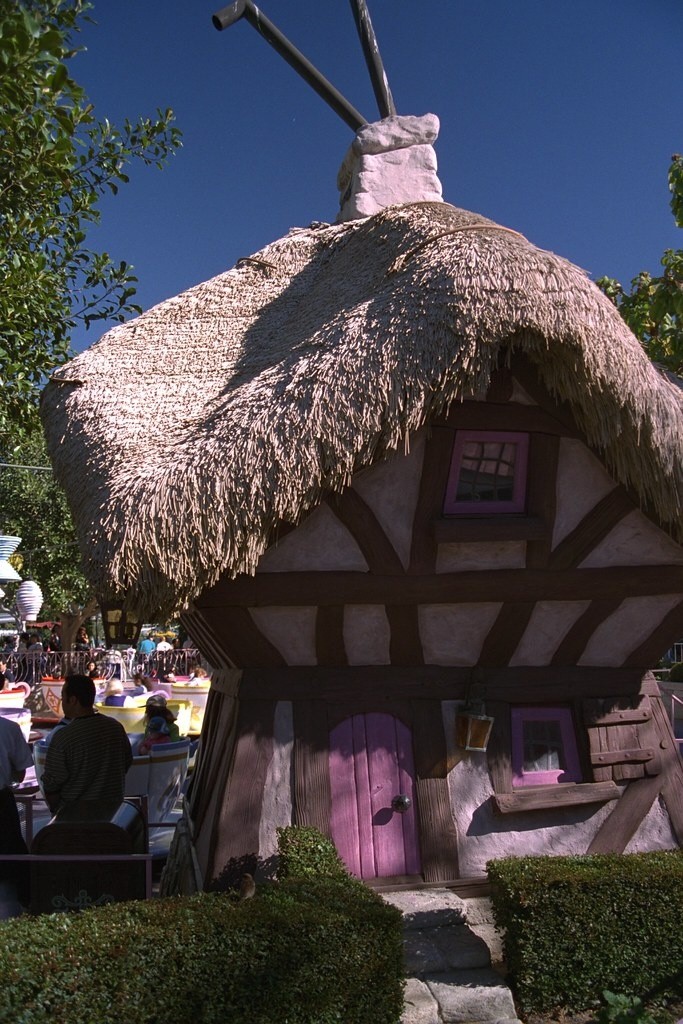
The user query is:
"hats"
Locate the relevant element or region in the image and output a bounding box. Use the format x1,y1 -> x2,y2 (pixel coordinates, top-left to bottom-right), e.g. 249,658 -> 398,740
138,695 -> 167,707
146,717 -> 170,734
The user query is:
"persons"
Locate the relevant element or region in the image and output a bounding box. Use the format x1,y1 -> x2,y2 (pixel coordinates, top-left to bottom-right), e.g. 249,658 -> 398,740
151,665 -> 177,682
139,695 -> 179,755
0,624 -> 63,685
105,678 -> 137,707
133,674 -> 153,695
75,627 -> 90,660
137,635 -> 195,673
189,664 -> 206,680
0,658 -> 16,690
35,675 -> 133,818
0,671 -> 34,904
87,661 -> 99,678
